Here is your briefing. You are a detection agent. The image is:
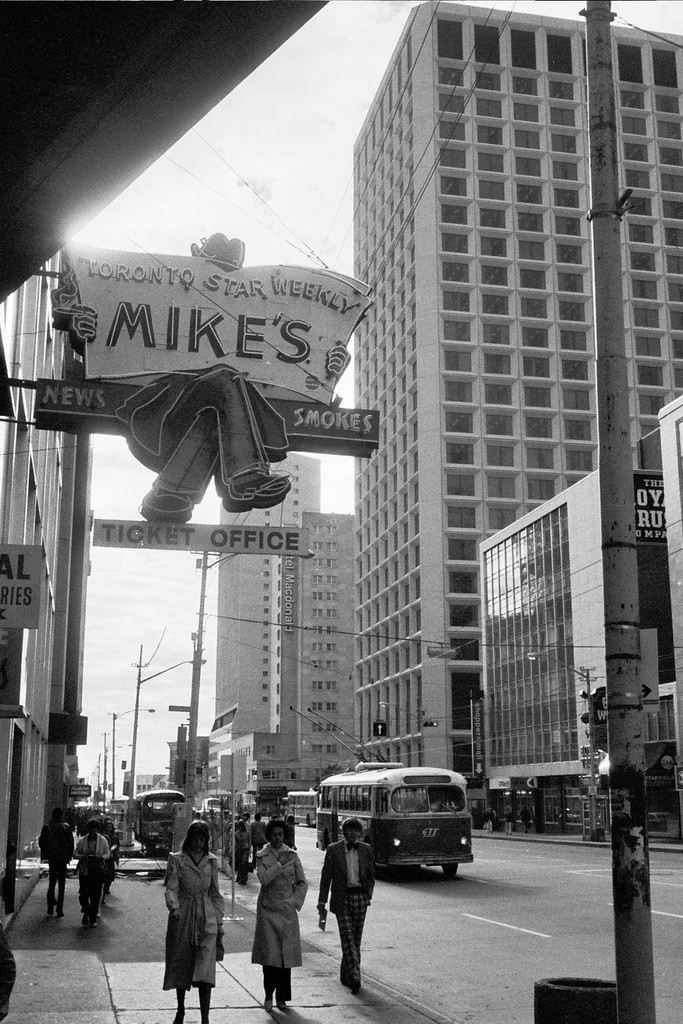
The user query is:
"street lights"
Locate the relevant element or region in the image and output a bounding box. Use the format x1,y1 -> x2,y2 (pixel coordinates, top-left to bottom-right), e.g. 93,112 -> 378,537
125,660 -> 208,846
528,653 -> 596,842
112,709 -> 155,800
104,744 -> 133,812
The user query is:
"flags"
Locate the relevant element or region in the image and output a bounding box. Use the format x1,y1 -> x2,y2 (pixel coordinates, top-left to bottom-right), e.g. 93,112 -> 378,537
644,742 -> 677,781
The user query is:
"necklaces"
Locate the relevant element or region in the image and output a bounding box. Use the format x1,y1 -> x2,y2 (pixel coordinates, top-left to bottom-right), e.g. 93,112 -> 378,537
87,833 -> 98,854
88,833 -> 97,842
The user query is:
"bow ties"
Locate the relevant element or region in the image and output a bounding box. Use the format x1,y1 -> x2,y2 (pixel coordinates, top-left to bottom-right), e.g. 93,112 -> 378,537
346,843 -> 359,851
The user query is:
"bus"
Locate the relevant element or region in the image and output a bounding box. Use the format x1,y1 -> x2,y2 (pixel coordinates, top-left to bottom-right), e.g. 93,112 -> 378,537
134,790 -> 185,844
286,791 -> 317,828
313,762 -> 474,880
203,798 -> 221,811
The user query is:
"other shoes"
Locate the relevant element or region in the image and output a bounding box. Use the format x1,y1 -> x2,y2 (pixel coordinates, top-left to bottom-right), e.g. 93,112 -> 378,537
88,920 -> 99,930
340,973 -> 361,995
47,909 -> 64,917
202,1013 -> 208,1024
264,999 -> 273,1012
173,1010 -> 185,1024
276,999 -> 289,1012
82,914 -> 88,926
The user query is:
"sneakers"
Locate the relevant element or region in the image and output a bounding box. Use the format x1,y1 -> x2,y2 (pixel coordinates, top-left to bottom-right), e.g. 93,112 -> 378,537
234,878 -> 247,885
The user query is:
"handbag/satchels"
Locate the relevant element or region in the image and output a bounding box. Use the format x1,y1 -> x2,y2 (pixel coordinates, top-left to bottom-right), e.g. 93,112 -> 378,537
78,854 -> 105,882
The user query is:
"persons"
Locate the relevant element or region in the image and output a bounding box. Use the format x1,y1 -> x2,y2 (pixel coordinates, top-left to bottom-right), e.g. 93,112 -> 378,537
0,920 -> 17,1021
436,789 -> 455,811
191,800 -> 297,884
317,819 -> 374,994
472,804 -> 531,835
38,806 -> 120,927
163,821 -> 225,1024
252,820 -> 308,1012
